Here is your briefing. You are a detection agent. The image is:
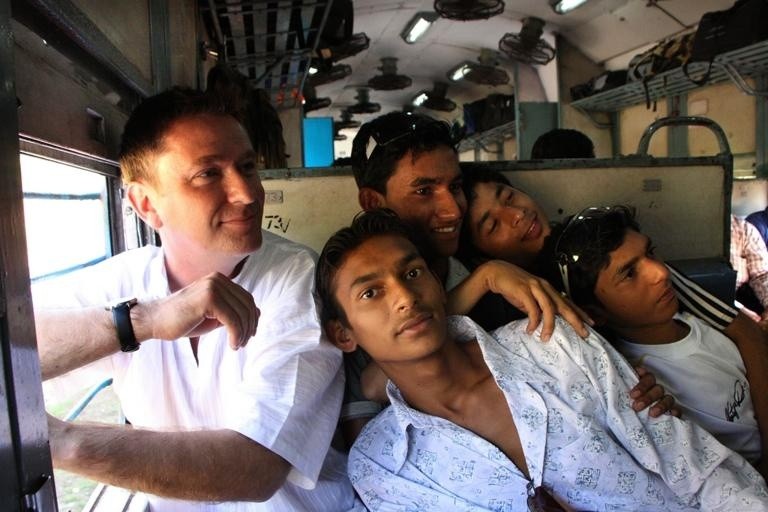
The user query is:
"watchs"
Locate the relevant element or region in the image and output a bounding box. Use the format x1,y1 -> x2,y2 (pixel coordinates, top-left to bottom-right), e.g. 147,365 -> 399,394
104,297 -> 140,354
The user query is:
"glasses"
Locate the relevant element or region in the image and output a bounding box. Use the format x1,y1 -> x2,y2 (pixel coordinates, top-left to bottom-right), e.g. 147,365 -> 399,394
553,204 -> 613,300
526,479 -> 565,511
364,114 -> 417,161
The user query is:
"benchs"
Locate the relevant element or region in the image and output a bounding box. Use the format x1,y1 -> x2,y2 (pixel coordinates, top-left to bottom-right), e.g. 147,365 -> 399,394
147,114 -> 739,314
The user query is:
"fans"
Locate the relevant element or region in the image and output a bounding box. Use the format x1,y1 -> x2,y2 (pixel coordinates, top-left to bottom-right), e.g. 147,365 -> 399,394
298,1 -> 560,146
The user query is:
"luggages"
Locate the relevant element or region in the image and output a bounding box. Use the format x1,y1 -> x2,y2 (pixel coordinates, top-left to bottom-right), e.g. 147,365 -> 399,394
570,70 -> 627,101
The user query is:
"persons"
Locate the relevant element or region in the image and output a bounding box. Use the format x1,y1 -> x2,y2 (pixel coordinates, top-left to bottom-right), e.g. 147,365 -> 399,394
315,207 -> 768,512
744,206 -> 768,250
35,84 -> 368,512
531,129 -> 596,158
454,159 -> 768,464
541,206 -> 768,485
341,109 -> 682,446
729,212 -> 768,320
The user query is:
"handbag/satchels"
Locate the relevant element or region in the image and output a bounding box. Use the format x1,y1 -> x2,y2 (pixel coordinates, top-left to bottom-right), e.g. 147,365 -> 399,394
627,32 -> 695,82
693,0 -> 768,61
461,91 -> 518,140
